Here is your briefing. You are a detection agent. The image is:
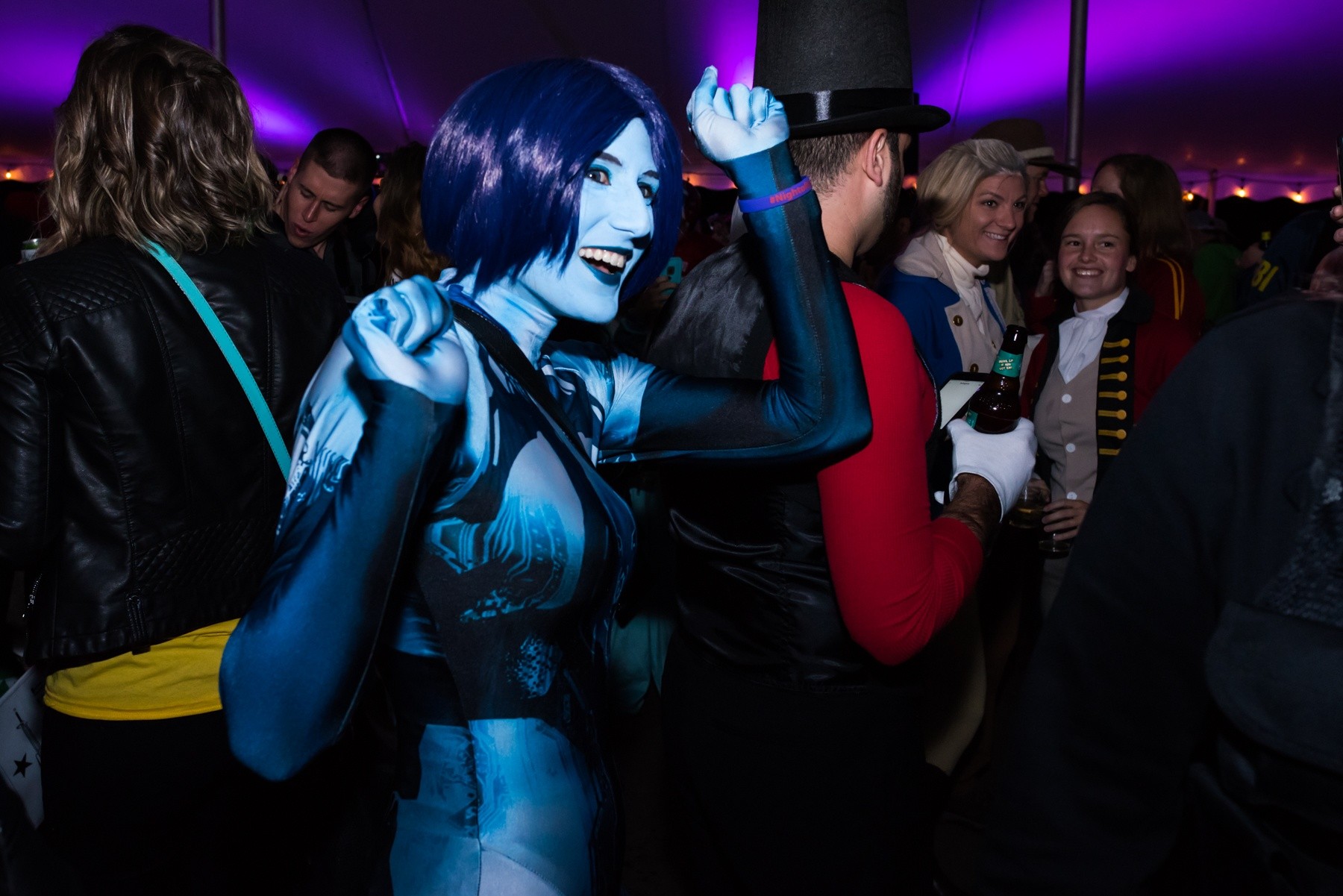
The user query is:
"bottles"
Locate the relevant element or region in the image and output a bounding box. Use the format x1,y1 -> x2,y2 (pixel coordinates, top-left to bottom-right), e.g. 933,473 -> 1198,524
965,325 -> 1029,434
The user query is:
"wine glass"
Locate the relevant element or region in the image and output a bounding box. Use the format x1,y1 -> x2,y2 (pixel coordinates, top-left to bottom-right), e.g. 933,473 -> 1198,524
1038,504 -> 1074,552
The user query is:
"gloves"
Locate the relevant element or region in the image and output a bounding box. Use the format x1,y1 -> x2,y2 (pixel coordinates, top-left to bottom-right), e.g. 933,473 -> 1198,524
945,417 -> 1038,525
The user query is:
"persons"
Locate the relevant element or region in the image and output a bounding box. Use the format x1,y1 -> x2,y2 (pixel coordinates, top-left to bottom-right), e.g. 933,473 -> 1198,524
371,141 -> 455,289
0,18 -> 414,896
265,127 -> 382,306
990,297 -> 1343,895
971,115 -> 1056,228
879,137 -> 1040,392
1195,102 -> 1342,306
1018,148 -> 1211,411
1014,188 -> 1200,633
215,44 -> 878,896
610,2 -> 1058,896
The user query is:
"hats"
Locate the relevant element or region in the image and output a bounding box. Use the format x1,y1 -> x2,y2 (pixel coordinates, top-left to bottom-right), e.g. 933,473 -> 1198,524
753,0 -> 953,139
972,116 -> 1083,180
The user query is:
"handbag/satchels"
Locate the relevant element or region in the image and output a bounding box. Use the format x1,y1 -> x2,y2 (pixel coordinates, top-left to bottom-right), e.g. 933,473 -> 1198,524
0,664 -> 45,831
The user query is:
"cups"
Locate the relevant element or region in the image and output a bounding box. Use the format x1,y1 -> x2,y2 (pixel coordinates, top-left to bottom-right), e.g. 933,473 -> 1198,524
1010,488 -> 1045,528
